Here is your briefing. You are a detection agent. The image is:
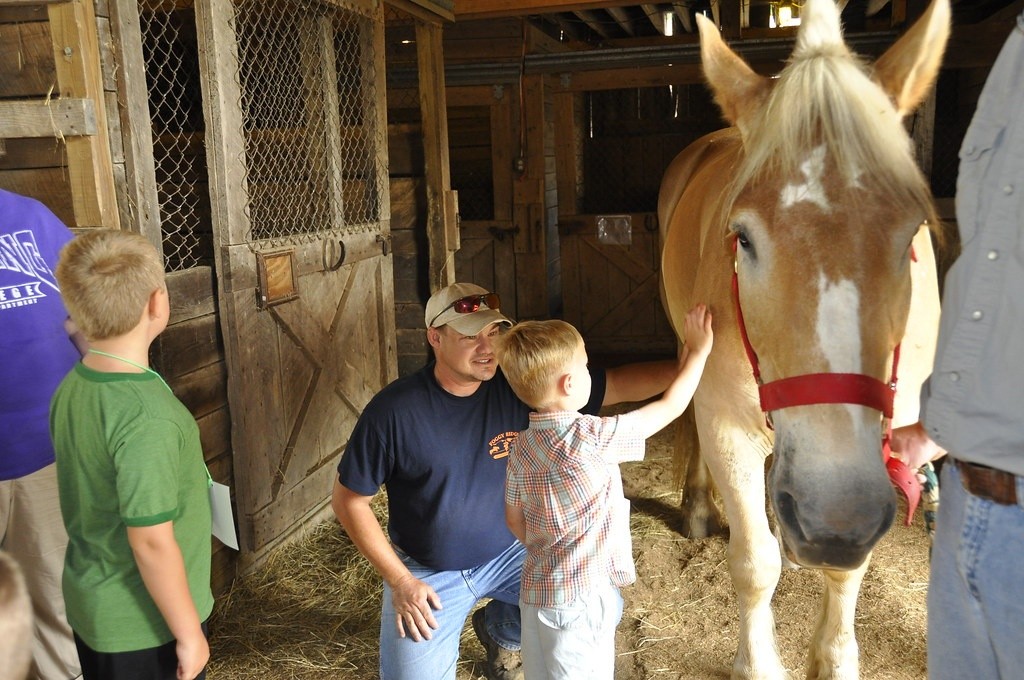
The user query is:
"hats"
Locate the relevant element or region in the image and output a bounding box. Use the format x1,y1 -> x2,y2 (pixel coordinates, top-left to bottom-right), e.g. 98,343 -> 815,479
424,284 -> 512,337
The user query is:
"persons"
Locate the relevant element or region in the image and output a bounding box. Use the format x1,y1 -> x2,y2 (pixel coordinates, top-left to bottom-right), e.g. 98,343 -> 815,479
330,282 -> 691,679
491,303 -> 713,680
0,551 -> 36,679
0,190 -> 87,679
920,0 -> 1024,680
42,229 -> 216,680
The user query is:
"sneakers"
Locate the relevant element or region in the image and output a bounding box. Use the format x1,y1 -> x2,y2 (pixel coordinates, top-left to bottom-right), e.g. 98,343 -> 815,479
471,608 -> 525,680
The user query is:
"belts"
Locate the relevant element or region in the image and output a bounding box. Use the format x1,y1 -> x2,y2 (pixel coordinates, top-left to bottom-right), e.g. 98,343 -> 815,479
951,459 -> 1017,506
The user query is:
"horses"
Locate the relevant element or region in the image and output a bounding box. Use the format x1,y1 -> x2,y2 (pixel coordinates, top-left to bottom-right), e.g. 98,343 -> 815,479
656,1 -> 954,680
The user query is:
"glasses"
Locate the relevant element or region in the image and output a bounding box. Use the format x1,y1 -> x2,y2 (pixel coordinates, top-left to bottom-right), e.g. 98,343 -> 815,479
430,293 -> 500,328
